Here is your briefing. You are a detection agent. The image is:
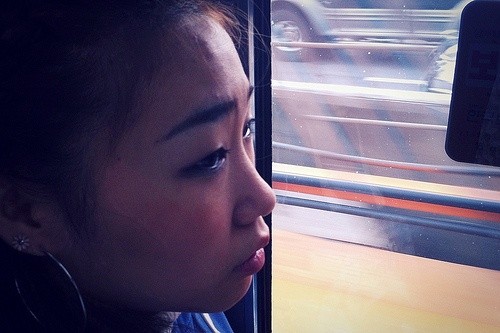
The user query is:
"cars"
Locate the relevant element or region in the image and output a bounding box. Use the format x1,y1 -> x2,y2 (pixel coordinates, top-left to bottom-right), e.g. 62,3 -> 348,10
271,1 -> 473,60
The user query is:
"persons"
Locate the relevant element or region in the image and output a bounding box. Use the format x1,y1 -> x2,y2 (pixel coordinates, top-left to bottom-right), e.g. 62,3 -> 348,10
1,0 -> 276,331
